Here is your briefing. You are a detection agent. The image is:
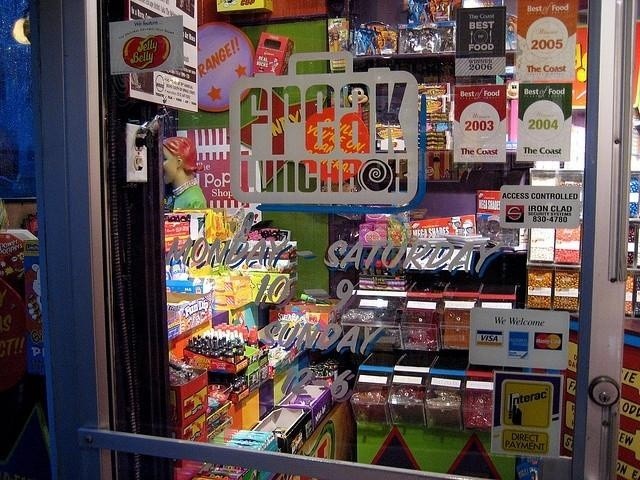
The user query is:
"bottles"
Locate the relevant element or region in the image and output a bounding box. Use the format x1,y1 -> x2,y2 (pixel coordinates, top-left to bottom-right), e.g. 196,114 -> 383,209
187,322 -> 259,363
212,372 -> 247,391
